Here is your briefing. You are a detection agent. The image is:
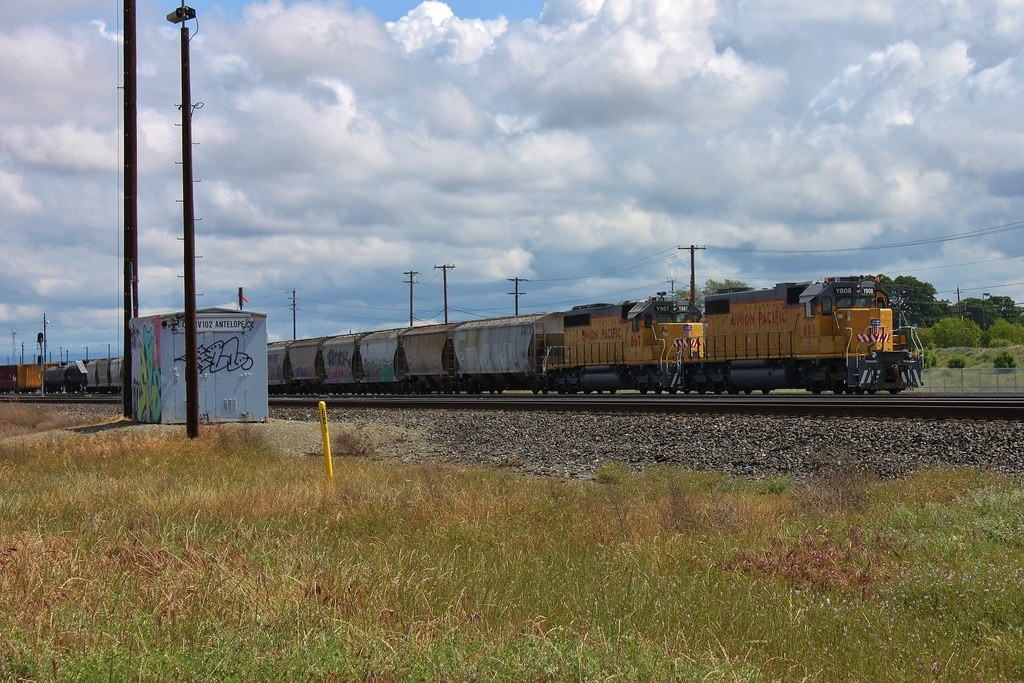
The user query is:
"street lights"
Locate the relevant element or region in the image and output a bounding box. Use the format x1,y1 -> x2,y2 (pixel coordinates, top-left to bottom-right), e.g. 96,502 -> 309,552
164,5 -> 199,441
40,313 -> 50,396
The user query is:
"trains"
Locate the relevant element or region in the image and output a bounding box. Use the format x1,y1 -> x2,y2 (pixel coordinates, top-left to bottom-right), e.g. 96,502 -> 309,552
1,273 -> 927,397
